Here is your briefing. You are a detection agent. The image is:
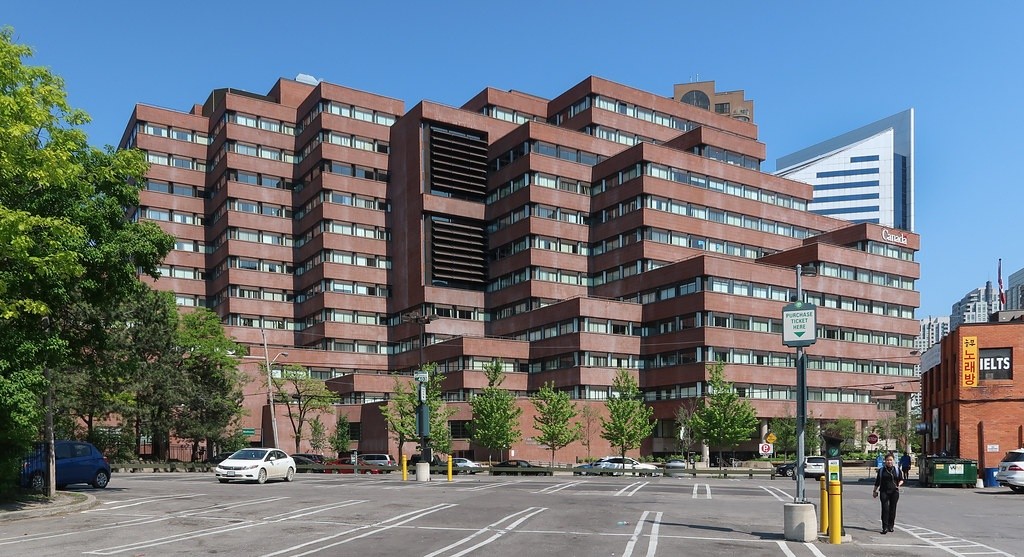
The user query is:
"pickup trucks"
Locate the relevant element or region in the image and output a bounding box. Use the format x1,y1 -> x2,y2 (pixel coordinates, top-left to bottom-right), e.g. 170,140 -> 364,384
406,453 -> 461,475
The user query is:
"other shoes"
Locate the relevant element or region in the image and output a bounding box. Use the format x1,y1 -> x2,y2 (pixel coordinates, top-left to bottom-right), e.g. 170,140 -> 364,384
890,526 -> 893,532
883,525 -> 887,533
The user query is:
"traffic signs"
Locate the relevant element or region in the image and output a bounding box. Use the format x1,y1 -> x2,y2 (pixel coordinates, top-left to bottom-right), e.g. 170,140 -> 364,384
781,300 -> 822,355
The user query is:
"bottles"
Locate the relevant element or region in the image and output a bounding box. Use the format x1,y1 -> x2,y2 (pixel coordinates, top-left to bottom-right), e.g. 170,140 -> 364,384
617,521 -> 629,525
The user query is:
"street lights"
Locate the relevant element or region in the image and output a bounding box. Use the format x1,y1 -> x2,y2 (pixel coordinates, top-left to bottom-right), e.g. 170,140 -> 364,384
794,263 -> 819,503
261,329 -> 289,450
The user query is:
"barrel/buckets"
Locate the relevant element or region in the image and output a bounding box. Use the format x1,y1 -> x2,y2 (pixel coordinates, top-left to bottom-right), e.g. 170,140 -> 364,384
984,467 -> 1000,488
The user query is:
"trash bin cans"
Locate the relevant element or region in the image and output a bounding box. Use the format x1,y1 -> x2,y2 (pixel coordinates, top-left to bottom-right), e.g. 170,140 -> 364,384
918,455 -> 939,484
983,467 -> 1002,487
416,462 -> 430,482
927,458 -> 977,489
875,456 -> 883,467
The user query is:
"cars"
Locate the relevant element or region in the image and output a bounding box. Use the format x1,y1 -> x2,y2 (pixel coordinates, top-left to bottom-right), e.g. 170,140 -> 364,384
325,458 -> 383,473
19,439 -> 112,490
290,454 -> 325,474
662,459 -> 687,469
574,460 -> 594,475
447,458 -> 484,473
204,452 -> 235,465
775,458 -> 798,477
492,459 -> 546,477
215,448 -> 296,483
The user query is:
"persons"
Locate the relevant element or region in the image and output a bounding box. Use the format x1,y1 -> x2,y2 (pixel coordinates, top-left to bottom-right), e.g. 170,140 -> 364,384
873,454 -> 903,534
899,452 -> 910,480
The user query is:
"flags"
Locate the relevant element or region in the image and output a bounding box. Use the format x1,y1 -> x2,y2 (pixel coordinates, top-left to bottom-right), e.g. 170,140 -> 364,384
998,260 -> 1006,304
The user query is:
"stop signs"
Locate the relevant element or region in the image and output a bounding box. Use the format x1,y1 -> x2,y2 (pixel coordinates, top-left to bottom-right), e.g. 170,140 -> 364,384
866,434 -> 879,445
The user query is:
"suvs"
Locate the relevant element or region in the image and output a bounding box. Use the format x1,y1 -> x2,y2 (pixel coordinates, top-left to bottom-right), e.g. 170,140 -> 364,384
593,456 -> 659,475
357,454 -> 398,472
995,448 -> 1024,493
793,455 -> 826,481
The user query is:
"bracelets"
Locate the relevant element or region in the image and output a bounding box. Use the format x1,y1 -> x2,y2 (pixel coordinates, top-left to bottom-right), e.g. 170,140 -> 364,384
873,491 -> 876,492
